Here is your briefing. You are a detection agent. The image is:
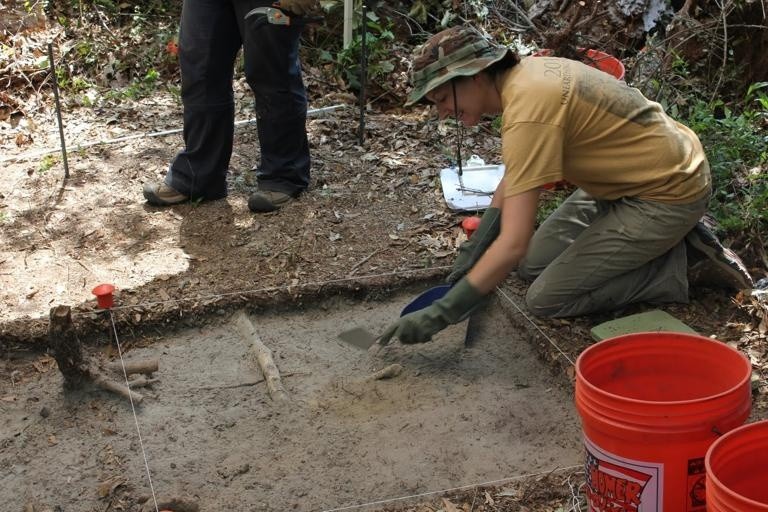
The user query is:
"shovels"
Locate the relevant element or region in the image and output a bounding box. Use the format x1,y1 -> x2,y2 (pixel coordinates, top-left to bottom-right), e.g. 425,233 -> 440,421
338,326 -> 384,351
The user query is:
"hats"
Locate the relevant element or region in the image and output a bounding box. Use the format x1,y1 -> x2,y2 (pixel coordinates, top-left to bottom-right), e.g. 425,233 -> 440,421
403,25 -> 508,106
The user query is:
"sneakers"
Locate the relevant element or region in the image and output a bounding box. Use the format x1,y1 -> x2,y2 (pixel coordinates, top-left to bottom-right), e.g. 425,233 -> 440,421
685,214 -> 754,289
247,190 -> 291,213
143,182 -> 189,206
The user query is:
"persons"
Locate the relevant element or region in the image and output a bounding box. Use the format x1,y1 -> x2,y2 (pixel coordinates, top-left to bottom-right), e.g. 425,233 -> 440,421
379,25 -> 758,347
143,0 -> 313,212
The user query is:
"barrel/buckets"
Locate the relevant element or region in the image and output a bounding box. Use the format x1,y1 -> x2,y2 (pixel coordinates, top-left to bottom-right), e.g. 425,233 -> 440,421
705,420 -> 768,512
530,47 -> 627,191
574,330 -> 751,511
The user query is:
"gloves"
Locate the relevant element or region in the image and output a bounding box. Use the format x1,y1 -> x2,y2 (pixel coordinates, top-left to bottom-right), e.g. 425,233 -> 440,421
380,207 -> 501,347
280,0 -> 313,16
